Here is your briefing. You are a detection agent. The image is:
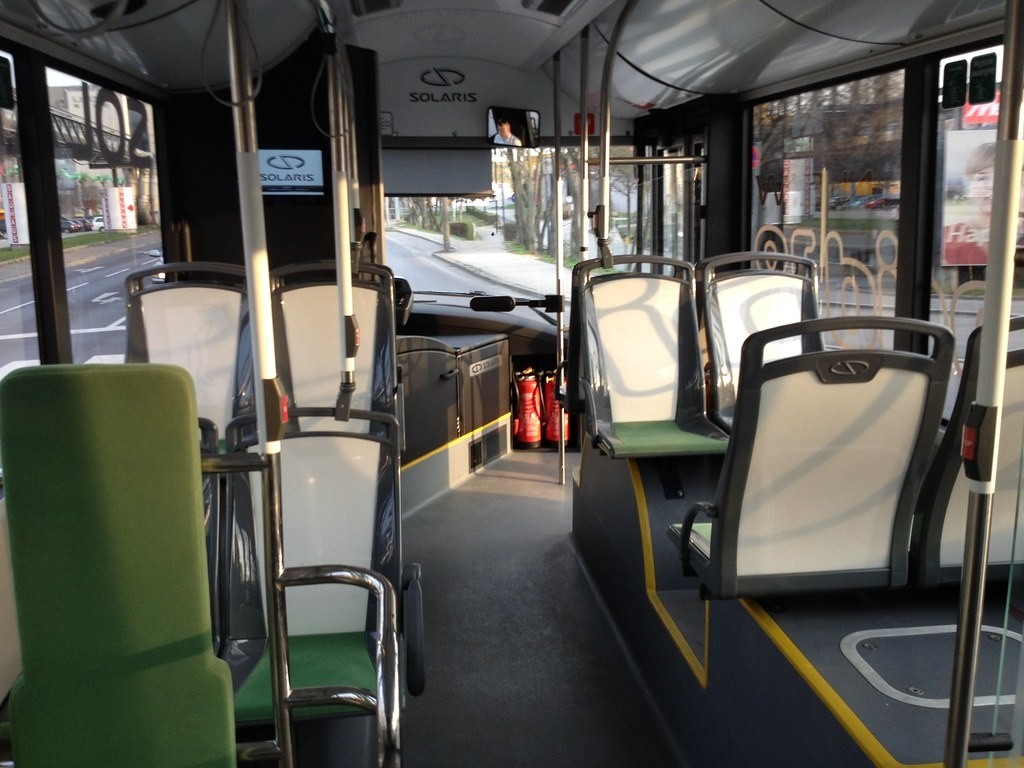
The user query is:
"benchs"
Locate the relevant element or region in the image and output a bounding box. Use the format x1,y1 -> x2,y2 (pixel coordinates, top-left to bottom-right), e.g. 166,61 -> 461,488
126,261 -> 426,728
668,317 -> 1024,602
556,251 -> 825,457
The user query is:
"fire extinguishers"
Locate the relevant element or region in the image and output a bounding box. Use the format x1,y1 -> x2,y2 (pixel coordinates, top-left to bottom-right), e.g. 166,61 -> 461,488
511,366 -> 543,450
538,369 -> 568,448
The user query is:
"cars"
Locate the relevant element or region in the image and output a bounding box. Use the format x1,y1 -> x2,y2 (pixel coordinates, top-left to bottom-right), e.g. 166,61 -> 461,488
59,214 -> 106,234
815,192 -> 900,212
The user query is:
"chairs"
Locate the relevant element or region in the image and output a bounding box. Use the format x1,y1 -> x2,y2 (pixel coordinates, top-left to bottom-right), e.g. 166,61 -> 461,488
0,364 -> 236,768
510,125 -> 525,147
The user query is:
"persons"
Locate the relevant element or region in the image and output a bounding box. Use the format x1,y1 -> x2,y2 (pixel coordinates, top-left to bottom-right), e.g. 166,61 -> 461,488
493,119 -> 522,147
944,142 -> 997,265
